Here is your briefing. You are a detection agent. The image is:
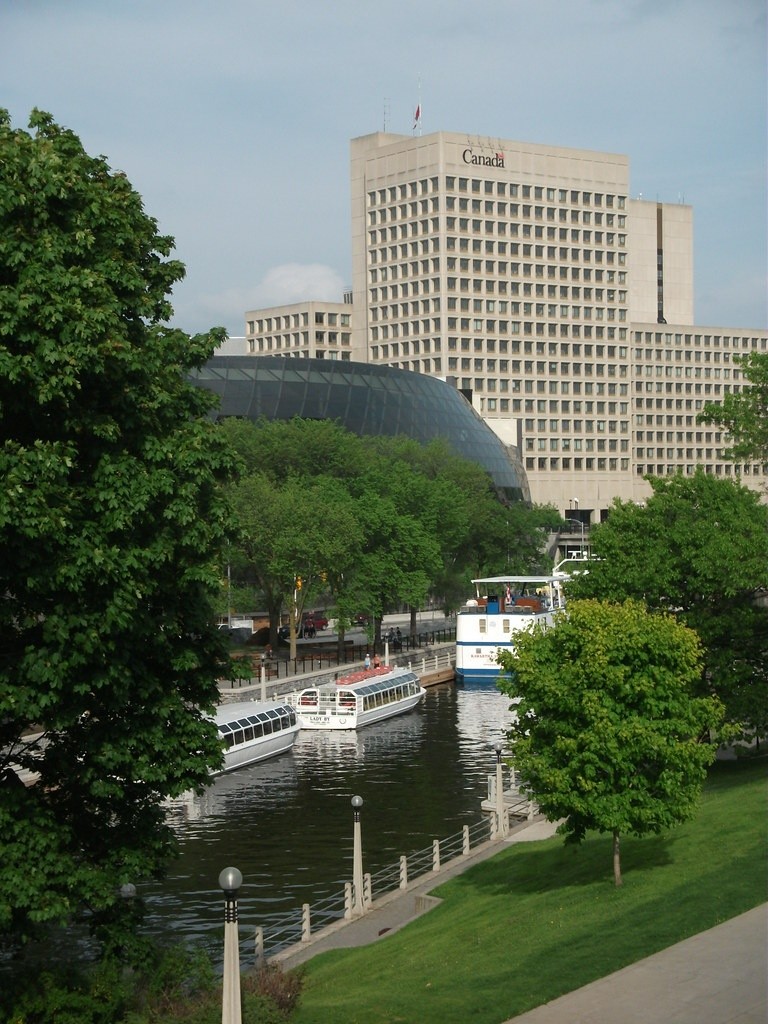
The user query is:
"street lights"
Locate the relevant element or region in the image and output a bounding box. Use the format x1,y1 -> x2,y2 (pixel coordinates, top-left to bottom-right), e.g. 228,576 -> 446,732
350,795 -> 364,913
260,653 -> 267,701
566,518 -> 584,559
217,866 -> 244,1024
493,742 -> 503,839
381,634 -> 392,666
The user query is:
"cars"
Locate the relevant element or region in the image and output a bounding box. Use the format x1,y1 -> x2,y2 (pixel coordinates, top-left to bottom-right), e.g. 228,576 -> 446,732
278,620 -> 318,639
302,613 -> 329,630
349,611 -> 370,626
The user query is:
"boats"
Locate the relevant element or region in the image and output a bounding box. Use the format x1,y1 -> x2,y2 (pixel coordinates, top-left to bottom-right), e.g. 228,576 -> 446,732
185,700 -> 304,780
455,575 -> 570,677
294,665 -> 427,730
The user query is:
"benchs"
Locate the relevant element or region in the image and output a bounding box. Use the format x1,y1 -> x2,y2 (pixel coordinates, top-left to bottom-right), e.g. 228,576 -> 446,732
230,655 -> 278,677
298,640 -> 355,660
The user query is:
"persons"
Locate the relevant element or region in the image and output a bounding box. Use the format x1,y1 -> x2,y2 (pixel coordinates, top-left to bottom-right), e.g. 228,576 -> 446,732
364,654 -> 371,671
389,627 -> 401,637
374,654 -> 380,669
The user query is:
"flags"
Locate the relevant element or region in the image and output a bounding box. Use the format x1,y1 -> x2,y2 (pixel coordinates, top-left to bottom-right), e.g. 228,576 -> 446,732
412,104 -> 421,129
